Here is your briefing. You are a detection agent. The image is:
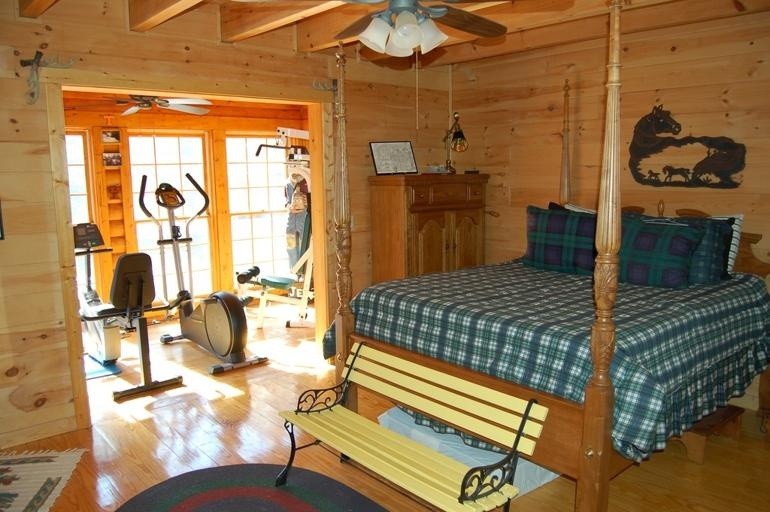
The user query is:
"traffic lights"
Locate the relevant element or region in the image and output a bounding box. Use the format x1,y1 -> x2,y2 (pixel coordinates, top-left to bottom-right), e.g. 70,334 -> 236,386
522,201 -> 744,289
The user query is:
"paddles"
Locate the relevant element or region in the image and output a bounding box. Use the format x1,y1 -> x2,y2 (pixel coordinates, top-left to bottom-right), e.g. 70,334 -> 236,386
0,447 -> 87,512
111,464 -> 389,512
377,406 -> 564,501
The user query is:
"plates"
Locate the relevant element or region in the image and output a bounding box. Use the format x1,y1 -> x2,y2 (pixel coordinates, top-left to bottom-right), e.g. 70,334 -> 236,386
368,173 -> 490,286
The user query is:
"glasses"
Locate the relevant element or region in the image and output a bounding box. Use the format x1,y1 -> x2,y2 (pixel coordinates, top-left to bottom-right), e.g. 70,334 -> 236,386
359,0 -> 450,58
442,112 -> 468,174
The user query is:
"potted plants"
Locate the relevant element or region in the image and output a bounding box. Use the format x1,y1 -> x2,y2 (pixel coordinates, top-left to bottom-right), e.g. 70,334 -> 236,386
369,140 -> 418,175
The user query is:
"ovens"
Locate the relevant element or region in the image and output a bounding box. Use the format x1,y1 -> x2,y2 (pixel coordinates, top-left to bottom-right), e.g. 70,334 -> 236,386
346,207 -> 769,512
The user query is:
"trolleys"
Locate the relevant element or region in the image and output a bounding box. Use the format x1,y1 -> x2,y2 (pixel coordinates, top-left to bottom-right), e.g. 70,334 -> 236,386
116,93 -> 214,116
333,0 -> 508,40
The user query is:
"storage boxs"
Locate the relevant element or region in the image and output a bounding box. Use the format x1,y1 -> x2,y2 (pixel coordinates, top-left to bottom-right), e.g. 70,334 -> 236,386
275,342 -> 548,511
